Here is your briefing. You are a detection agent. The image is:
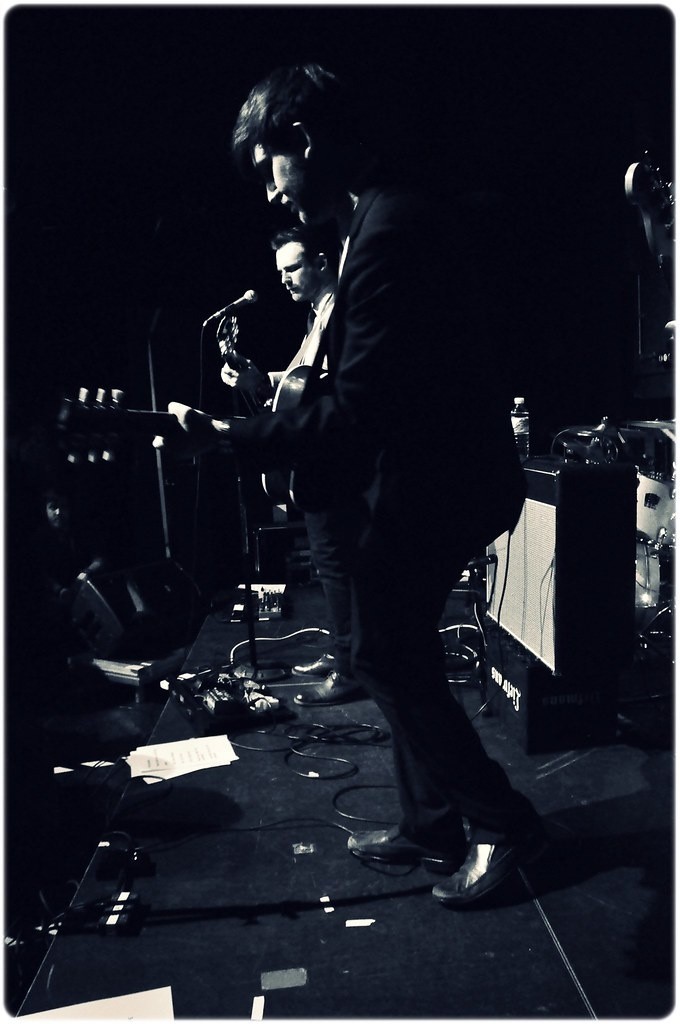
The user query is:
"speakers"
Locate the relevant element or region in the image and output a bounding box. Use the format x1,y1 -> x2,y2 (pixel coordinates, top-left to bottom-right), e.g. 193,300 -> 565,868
485,455 -> 636,680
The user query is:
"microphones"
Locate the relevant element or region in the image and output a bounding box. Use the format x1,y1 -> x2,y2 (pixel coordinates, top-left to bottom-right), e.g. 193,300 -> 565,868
466,554 -> 497,568
202,289 -> 258,327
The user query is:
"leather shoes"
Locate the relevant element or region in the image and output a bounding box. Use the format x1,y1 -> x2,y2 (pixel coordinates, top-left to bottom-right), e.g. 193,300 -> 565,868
294,670 -> 369,705
291,651 -> 335,677
433,824 -> 549,907
347,826 -> 455,871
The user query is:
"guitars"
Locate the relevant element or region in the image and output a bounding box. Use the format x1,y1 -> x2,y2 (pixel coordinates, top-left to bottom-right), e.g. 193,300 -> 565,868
218,335 -> 275,416
625,149 -> 675,372
58,364 -> 333,515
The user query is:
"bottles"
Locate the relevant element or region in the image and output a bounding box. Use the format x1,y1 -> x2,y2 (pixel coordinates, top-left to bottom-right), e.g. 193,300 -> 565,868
511,397 -> 530,458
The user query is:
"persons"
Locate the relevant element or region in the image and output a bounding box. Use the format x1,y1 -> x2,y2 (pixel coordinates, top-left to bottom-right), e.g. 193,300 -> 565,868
38,491 -> 115,709
190,64 -> 550,907
